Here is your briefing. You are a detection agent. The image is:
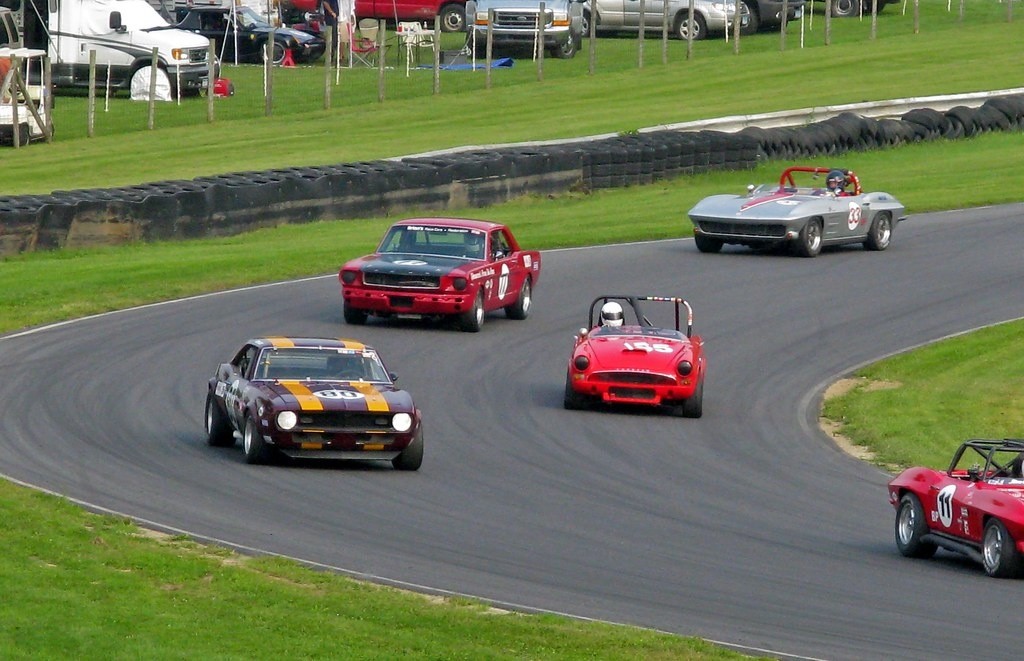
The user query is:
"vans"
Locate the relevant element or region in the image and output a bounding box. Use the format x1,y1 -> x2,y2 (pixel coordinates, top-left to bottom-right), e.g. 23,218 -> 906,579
0,0 -> 219,103
472,0 -> 586,59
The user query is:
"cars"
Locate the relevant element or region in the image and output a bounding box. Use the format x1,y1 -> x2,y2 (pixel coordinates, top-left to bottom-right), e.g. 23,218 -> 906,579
173,6 -> 326,66
340,218 -> 541,331
563,293 -> 706,418
580,0 -> 750,41
886,439 -> 1024,580
203,333 -> 426,468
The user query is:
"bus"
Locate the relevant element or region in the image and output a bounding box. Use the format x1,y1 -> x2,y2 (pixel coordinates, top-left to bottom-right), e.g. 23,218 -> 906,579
288,0 -> 467,33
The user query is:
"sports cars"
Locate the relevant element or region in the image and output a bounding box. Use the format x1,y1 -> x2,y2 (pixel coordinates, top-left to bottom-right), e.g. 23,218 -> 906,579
687,165 -> 909,258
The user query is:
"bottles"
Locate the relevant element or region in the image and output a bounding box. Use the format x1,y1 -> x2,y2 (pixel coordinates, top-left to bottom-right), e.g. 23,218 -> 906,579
423,21 -> 427,32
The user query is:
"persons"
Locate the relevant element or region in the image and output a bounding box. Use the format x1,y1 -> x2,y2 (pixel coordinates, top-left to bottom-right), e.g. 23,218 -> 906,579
461,234 -> 496,261
338,0 -> 357,59
323,0 -> 340,61
826,171 -> 846,191
601,302 -> 624,327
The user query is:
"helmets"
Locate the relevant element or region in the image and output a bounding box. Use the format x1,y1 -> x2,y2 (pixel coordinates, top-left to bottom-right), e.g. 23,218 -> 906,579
601,302 -> 624,327
464,232 -> 485,254
826,170 -> 846,192
